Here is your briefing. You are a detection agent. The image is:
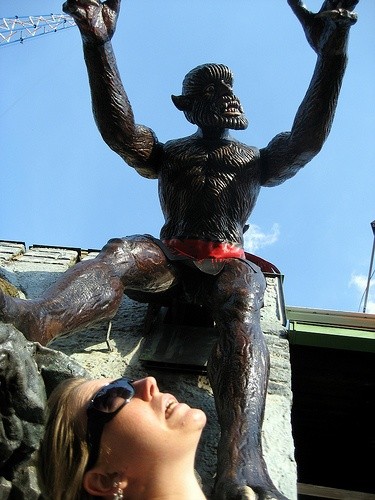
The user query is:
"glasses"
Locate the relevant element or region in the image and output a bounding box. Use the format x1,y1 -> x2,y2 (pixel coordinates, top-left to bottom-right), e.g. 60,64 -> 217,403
84,376 -> 139,475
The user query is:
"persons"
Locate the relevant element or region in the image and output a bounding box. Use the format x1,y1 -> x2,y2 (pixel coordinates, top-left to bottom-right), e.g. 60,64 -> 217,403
0,0 -> 362,499
41,375 -> 209,500
